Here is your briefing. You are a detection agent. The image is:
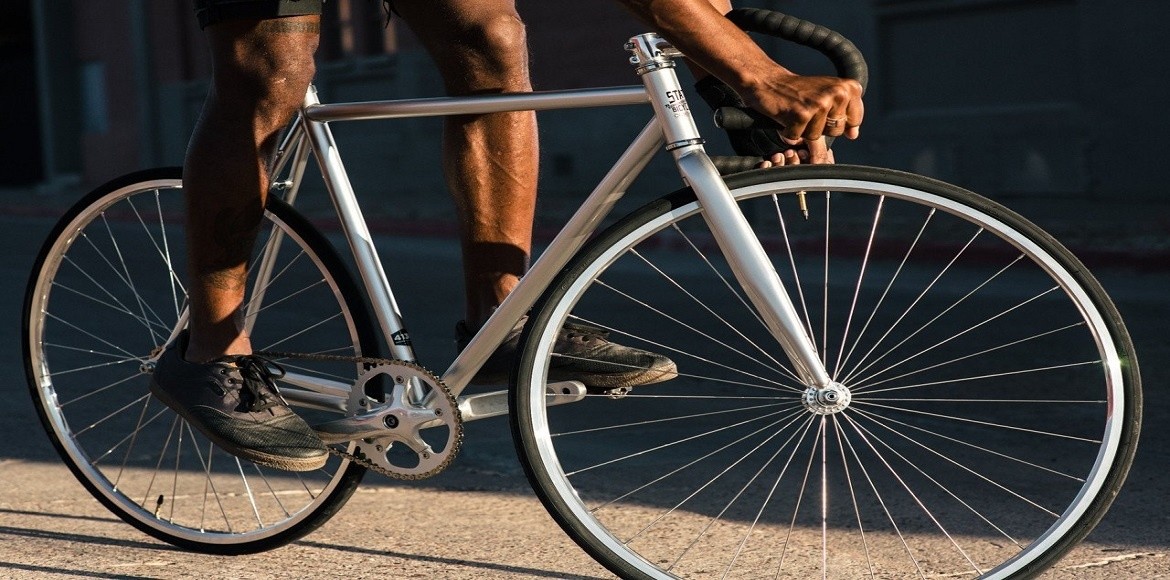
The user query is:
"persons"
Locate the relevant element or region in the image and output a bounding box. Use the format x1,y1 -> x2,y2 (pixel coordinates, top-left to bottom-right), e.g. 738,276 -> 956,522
152,0 -> 865,474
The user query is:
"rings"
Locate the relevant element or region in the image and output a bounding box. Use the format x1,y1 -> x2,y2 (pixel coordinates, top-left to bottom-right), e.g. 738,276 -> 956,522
827,117 -> 848,127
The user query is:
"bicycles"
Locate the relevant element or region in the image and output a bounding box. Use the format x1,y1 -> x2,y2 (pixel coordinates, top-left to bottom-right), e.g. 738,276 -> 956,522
23,9 -> 1146,578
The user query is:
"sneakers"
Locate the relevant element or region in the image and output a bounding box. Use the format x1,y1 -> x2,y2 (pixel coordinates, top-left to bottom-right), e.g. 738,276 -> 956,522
453,305 -> 678,389
148,329 -> 330,472
